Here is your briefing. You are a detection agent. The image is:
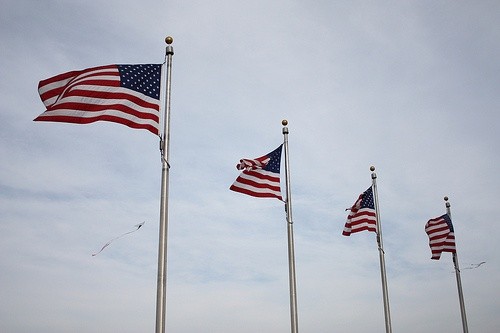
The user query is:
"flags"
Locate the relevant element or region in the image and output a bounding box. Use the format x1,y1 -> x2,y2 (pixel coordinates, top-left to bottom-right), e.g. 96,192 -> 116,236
425,213 -> 456,260
32,61 -> 166,142
228,141 -> 288,205
342,185 -> 378,236
